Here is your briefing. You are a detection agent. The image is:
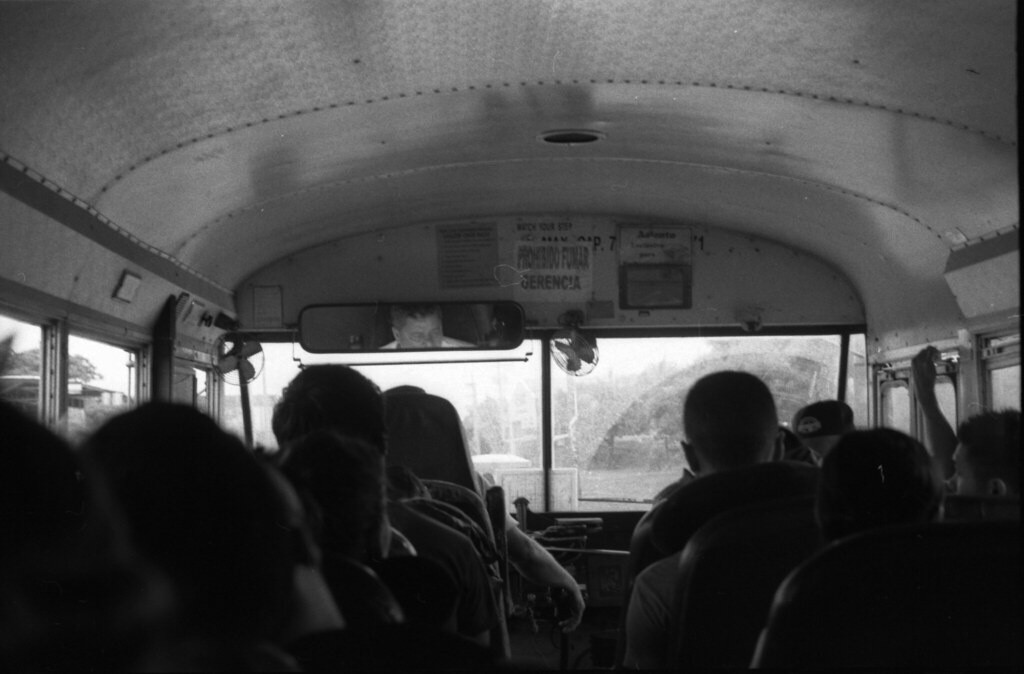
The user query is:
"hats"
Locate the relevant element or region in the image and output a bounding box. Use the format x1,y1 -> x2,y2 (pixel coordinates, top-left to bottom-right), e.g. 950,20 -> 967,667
792,401 -> 854,438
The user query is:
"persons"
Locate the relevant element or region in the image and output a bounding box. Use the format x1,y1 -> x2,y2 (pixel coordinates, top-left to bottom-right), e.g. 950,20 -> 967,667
0,363 -> 586,674
619,345 -> 1024,674
379,304 -> 475,349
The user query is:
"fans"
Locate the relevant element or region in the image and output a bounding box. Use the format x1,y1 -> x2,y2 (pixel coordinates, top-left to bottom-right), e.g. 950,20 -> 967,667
548,311 -> 598,377
210,330 -> 264,386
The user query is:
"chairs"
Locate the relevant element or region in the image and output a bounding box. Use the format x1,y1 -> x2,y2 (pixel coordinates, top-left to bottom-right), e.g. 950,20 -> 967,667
202,478 -> 1024,674
